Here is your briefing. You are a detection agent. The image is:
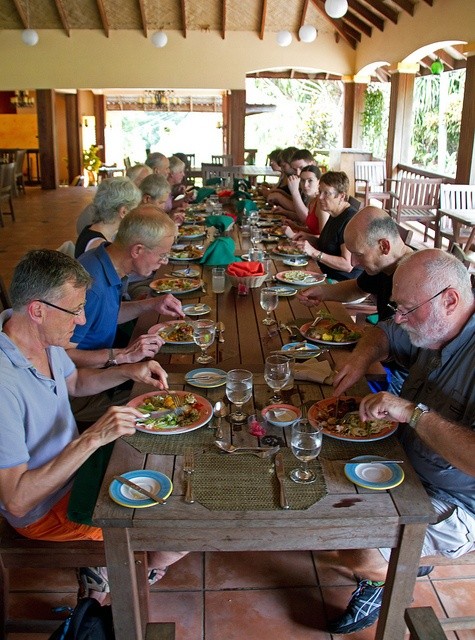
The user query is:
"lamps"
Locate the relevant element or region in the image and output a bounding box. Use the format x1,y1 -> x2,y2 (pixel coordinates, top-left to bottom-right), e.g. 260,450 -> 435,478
21,0 -> 39,46
10,89 -> 34,107
299,24 -> 317,42
137,90 -> 181,112
216,121 -> 222,129
325,0 -> 348,19
275,30 -> 292,47
150,30 -> 168,47
431,48 -> 444,74
84,118 -> 88,127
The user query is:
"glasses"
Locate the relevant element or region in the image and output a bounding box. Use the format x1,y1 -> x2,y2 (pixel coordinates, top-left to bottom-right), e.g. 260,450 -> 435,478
386,286 -> 451,317
36,300 -> 82,316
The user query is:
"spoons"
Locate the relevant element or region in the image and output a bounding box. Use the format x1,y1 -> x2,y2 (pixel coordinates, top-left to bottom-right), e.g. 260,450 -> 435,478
216,322 -> 226,344
283,323 -> 297,341
211,400 -> 226,439
212,440 -> 276,453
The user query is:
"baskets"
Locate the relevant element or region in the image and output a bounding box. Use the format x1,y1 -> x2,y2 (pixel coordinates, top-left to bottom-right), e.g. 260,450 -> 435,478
225,272 -> 268,289
219,196 -> 230,204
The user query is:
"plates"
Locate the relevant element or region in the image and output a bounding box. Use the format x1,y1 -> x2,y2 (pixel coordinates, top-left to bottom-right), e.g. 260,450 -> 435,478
182,216 -> 205,224
174,243 -> 204,251
344,455 -> 406,490
182,303 -> 210,315
299,319 -> 365,345
284,259 -> 308,267
170,244 -> 205,260
147,320 -> 204,344
307,396 -> 400,443
263,236 -> 278,241
272,244 -> 309,258
253,200 -> 266,203
259,214 -> 286,221
274,268 -> 327,286
279,342 -> 321,360
268,285 -> 297,298
184,368 -> 227,389
189,213 -> 207,217
149,278 -> 203,294
241,253 -> 270,260
171,269 -> 200,277
182,225 -> 204,229
179,229 -> 206,239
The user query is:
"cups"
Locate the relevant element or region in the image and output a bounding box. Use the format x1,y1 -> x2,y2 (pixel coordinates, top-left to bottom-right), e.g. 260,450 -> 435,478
248,415 -> 267,435
109,470 -> 172,510
212,203 -> 222,216
213,268 -> 225,295
242,218 -> 251,237
124,390 -> 215,435
272,352 -> 295,390
248,248 -> 263,263
258,258 -> 273,281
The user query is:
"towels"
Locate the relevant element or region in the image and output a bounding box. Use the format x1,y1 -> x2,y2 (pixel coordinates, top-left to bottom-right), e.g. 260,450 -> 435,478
200,237 -> 243,268
204,215 -> 234,235
205,177 -> 228,186
293,358 -> 331,384
225,213 -> 237,223
217,190 -> 234,197
230,190 -> 251,200
366,315 -> 379,325
236,198 -> 259,214
188,187 -> 216,204
233,178 -> 251,191
227,261 -> 264,277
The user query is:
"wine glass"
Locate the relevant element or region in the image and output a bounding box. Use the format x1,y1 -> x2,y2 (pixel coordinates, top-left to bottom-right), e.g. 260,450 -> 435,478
240,207 -> 248,232
215,184 -> 222,193
289,419 -> 323,485
249,211 -> 259,229
210,196 -> 218,215
259,289 -> 279,326
251,229 -> 264,248
193,319 -> 215,364
175,226 -> 181,244
264,356 -> 290,403
227,370 -> 253,424
238,195 -> 246,202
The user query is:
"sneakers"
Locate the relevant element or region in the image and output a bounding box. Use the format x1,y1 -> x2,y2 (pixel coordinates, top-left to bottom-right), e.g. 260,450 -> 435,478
416,566 -> 433,577
147,567 -> 167,586
334,579 -> 385,634
76,566 -> 111,604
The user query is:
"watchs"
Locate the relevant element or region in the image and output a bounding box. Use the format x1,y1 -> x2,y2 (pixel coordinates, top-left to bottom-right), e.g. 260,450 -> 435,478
409,402 -> 431,429
317,251 -> 323,262
104,348 -> 118,368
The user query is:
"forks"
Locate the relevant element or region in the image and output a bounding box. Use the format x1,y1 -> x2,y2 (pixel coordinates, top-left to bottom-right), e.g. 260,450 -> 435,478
306,298 -> 333,320
182,448 -> 196,504
164,388 -> 182,411
183,317 -> 193,325
294,273 -> 326,281
339,411 -> 361,424
208,448 -> 281,458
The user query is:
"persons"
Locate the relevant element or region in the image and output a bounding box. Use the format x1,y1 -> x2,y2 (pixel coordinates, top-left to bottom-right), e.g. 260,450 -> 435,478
291,170 -> 363,283
267,146 -> 300,212
167,156 -> 195,207
75,177 -> 144,258
296,205 -> 420,398
125,163 -> 152,187
63,204 -> 186,425
272,149 -> 317,224
0,247 -> 190,607
145,152 -> 170,179
280,165 -> 331,239
260,147 -> 287,197
138,173 -> 186,225
329,250 -> 474,636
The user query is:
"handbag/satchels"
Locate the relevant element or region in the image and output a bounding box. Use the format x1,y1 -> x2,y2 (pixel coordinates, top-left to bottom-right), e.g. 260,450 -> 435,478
49,597 -> 114,639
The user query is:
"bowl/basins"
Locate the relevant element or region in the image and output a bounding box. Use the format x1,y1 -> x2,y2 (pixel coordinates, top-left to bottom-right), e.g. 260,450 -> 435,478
261,404 -> 301,427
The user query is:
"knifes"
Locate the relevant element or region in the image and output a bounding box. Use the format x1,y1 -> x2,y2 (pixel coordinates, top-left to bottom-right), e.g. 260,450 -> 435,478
132,409 -> 170,421
183,376 -> 226,382
271,453 -> 292,509
115,474 -> 168,508
332,459 -> 405,465
305,316 -> 321,337
162,328 -> 174,339
269,348 -> 329,355
334,395 -> 340,420
289,347 -> 329,355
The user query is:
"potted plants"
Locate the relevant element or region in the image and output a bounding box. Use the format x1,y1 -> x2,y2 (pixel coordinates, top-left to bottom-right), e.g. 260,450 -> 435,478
83,145 -> 102,186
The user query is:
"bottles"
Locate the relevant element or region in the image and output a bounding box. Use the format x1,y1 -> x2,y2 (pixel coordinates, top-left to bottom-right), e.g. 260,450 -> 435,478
206,195 -> 213,213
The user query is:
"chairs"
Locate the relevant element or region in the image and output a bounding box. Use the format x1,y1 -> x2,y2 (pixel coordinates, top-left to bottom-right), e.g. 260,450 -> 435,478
186,154 -> 196,181
0,275 -> 12,313
348,195 -> 365,210
212,155 -> 224,165
406,551 -> 475,634
439,183 -> 475,252
218,165 -> 243,186
14,149 -> 27,196
344,221 -> 414,324
354,161 -> 400,212
0,162 -> 17,228
144,605 -> 447,639
201,163 -> 223,186
450,242 -> 470,271
0,515 -> 151,640
124,157 -> 130,169
390,177 -> 447,248
